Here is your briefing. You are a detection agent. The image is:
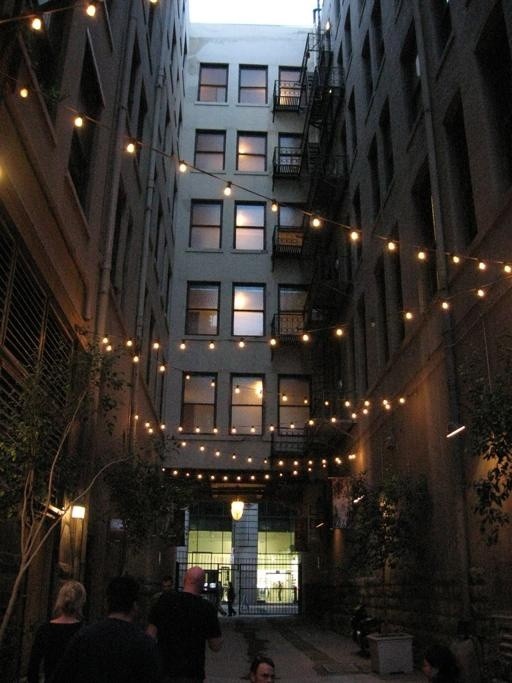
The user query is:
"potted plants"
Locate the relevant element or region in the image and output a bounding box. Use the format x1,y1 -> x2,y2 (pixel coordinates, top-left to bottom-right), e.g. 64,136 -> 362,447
330,459 -> 437,678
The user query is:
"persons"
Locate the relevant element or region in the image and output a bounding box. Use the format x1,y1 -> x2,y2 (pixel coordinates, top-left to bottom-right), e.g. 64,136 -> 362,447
145,565 -> 223,680
214,579 -> 238,616
352,594 -> 373,631
50,575 -> 159,680
248,654 -> 276,681
421,643 -> 460,681
25,579 -> 89,680
324,585 -> 338,625
149,574 -> 177,612
